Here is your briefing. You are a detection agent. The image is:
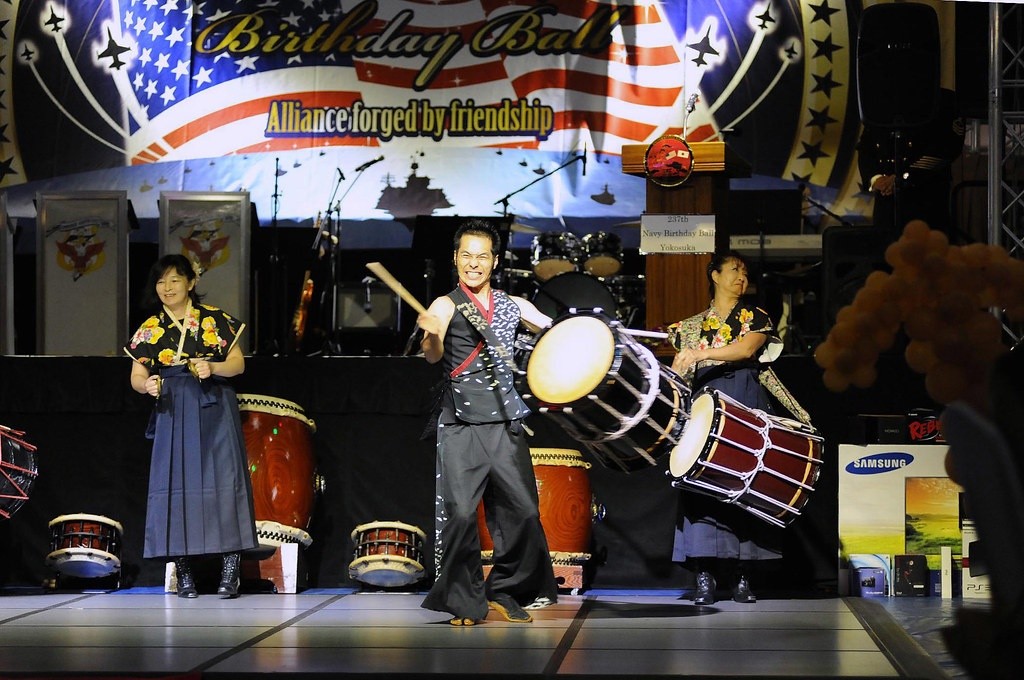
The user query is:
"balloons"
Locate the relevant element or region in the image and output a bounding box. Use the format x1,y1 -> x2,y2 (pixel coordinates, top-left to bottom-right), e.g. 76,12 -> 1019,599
814,221 -> 1024,404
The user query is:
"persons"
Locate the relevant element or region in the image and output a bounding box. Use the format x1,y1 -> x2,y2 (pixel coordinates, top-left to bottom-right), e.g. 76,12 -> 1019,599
128,254 -> 259,598
670,251 -> 783,602
415,221 -> 556,626
858,87 -> 966,248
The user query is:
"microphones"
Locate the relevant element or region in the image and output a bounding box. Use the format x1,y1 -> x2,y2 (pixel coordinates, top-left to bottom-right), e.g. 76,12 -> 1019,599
686,89 -> 701,113
799,184 -> 806,193
356,156 -> 384,171
363,286 -> 372,313
337,168 -> 345,180
583,142 -> 586,176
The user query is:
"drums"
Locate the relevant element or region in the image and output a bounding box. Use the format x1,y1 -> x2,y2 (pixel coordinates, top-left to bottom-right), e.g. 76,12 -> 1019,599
517,305 -> 694,477
504,268 -> 534,341
348,520 -> 428,588
530,229 -> 582,280
233,391 -> 319,550
44,512 -> 125,579
477,447 -> 596,566
0,426 -> 37,522
582,231 -> 624,277
532,270 -> 632,342
662,385 -> 827,530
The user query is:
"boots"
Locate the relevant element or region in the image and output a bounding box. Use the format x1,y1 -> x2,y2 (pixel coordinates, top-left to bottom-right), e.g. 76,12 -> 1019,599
695,565 -> 715,604
733,570 -> 757,603
218,554 -> 241,598
175,557 -> 198,598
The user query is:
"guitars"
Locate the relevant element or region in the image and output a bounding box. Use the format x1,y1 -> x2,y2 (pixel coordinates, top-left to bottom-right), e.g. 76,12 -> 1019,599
290,209 -> 323,340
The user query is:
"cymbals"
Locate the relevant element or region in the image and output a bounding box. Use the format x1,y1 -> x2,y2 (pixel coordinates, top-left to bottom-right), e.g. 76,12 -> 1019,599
612,219 -> 640,228
504,250 -> 519,261
493,210 -> 532,221
510,223 -> 540,235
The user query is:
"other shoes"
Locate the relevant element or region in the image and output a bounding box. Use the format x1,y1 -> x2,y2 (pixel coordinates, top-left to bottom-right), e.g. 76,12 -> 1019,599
488,593 -> 534,622
450,616 -> 476,625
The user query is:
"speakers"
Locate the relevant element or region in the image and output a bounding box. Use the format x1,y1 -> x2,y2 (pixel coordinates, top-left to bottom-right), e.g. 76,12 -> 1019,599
334,281 -> 400,334
823,223 -> 877,336
856,1 -> 941,131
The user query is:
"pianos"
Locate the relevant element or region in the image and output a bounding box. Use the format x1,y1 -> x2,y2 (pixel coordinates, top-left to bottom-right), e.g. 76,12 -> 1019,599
728,231 -> 824,359
